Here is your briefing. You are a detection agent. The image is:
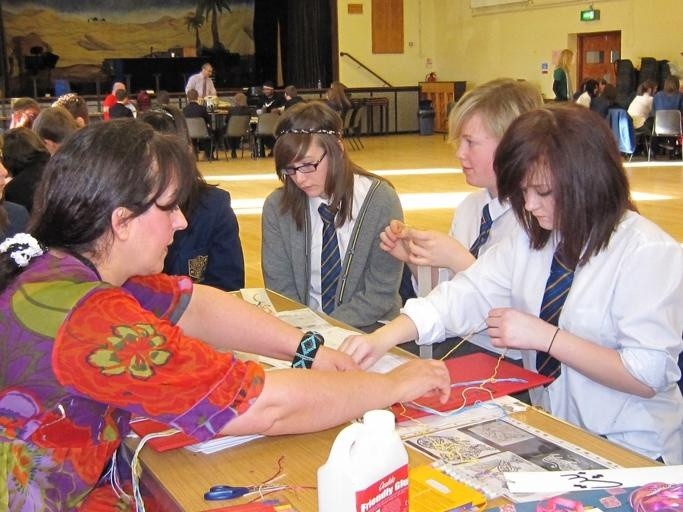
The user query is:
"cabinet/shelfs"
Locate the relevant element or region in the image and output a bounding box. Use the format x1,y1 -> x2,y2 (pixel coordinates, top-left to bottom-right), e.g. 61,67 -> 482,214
418,80 -> 467,133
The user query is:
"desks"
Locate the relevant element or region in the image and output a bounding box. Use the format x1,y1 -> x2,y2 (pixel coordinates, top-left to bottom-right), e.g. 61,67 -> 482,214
113,287 -> 683,511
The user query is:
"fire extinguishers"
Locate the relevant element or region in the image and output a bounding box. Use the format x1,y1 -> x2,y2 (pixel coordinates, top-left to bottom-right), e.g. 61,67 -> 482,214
425,72 -> 437,83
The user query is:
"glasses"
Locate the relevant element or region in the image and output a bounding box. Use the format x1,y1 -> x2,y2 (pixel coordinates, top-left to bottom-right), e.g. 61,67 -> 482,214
279,150 -> 329,175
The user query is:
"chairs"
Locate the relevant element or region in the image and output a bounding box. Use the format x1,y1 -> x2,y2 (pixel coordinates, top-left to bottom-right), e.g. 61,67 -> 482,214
606,107 -> 648,161
175,93 -> 369,162
645,109 -> 681,162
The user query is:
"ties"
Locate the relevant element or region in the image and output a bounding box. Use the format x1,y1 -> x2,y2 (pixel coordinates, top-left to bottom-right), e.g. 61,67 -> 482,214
470,205 -> 492,258
535,242 -> 581,387
202,78 -> 206,97
317,203 -> 342,317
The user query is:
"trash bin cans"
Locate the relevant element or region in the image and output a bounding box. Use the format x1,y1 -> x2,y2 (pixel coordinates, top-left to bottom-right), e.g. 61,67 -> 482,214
418,109 -> 435,135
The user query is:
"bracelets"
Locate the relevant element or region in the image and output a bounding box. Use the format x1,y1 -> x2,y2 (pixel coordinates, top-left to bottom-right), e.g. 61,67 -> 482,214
543,328 -> 558,353
291,329 -> 325,367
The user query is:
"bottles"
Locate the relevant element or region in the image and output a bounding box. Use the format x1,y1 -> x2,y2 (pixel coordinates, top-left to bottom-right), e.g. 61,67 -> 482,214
206,96 -> 213,113
317,79 -> 321,91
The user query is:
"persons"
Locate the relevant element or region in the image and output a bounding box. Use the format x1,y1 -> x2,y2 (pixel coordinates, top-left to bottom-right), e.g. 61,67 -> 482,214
0,94 -> 247,299
261,99 -> 403,335
377,77 -> 545,408
105,64 -> 349,158
551,47 -> 682,158
333,102 -> 682,466
0,118 -> 451,510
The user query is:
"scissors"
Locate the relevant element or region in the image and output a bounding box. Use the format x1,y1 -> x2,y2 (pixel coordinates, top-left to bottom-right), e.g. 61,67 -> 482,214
204,485 -> 289,500
